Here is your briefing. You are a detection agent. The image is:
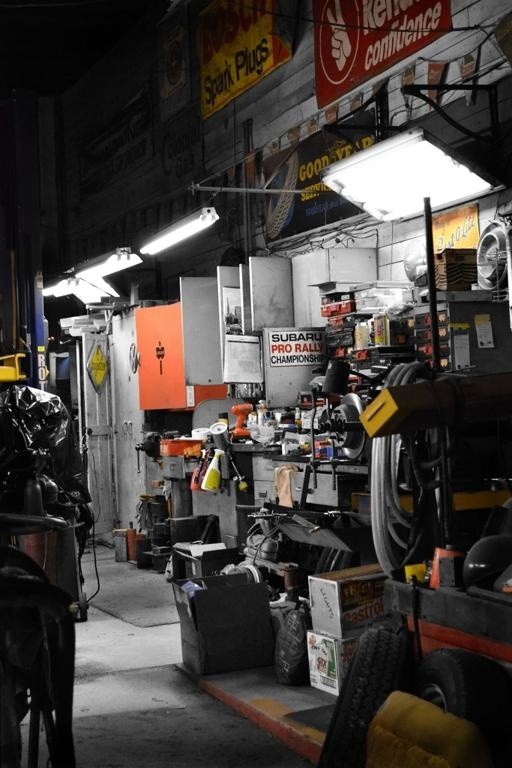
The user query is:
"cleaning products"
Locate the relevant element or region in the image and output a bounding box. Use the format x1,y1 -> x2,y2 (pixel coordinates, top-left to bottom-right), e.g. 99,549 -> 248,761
189,448 -> 212,490
200,449 -> 225,492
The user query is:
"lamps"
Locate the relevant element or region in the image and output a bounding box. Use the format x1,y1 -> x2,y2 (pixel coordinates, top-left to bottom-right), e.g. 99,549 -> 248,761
42,206 -> 224,303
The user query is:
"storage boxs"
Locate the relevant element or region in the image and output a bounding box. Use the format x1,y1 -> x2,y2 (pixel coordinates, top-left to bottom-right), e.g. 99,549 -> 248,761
169,538 -> 274,678
300,564 -> 406,697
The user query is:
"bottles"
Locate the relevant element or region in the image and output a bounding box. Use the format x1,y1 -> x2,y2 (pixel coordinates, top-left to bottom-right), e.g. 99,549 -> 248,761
216,411 -> 230,433
354,312 -> 388,350
